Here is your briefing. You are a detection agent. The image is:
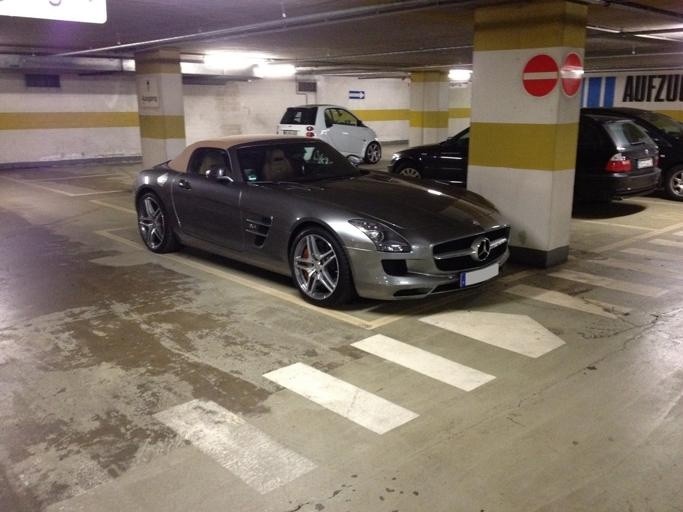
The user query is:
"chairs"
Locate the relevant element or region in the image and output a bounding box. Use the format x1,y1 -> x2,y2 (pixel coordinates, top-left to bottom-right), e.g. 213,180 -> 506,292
199,148 -> 296,182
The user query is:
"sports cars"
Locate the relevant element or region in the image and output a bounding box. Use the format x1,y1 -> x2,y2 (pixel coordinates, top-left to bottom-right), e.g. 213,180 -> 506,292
129,134 -> 513,310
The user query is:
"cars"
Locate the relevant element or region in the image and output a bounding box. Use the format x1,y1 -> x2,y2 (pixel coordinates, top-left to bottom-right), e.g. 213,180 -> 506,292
274,103 -> 384,164
386,111 -> 665,204
580,105 -> 683,202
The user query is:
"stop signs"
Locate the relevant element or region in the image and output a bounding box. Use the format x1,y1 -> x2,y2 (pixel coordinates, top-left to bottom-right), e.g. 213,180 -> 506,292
520,52 -> 559,99
559,48 -> 586,98
